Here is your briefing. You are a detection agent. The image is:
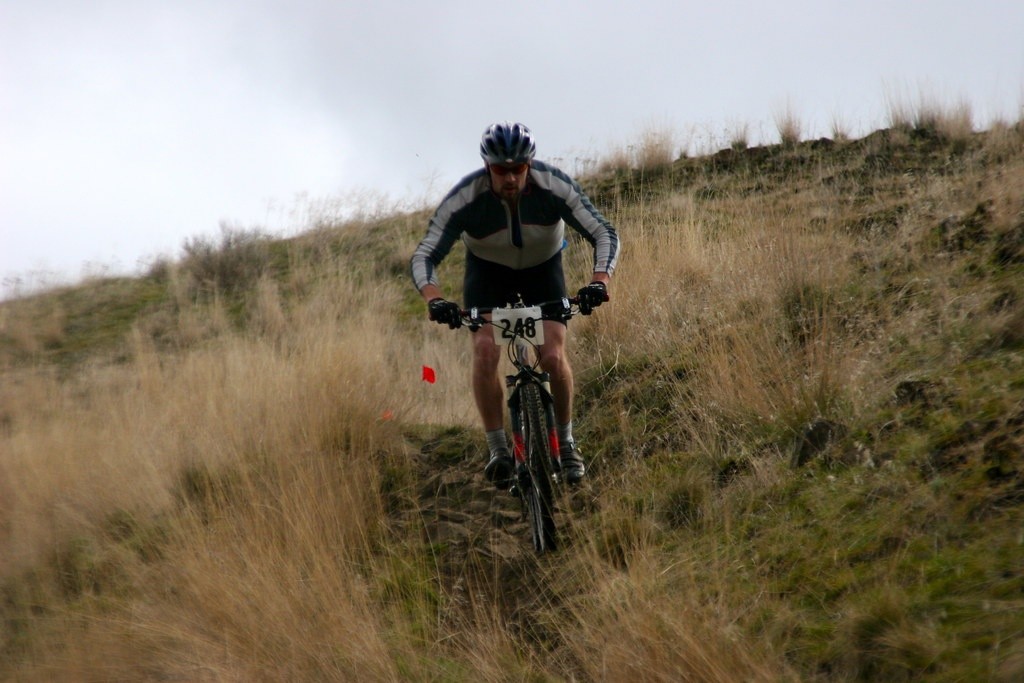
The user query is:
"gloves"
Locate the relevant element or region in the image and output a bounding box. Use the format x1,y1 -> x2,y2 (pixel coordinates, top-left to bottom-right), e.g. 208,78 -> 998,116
577,280 -> 606,316
429,298 -> 462,329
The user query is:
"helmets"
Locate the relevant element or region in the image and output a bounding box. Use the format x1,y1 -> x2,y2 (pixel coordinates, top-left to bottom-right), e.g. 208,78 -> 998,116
479,121 -> 536,165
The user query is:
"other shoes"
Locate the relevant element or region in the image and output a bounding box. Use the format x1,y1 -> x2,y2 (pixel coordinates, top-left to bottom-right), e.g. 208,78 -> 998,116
490,447 -> 513,491
561,442 -> 586,482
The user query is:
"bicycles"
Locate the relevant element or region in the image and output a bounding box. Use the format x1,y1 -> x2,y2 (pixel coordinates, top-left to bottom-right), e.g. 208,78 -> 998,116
426,283 -> 609,553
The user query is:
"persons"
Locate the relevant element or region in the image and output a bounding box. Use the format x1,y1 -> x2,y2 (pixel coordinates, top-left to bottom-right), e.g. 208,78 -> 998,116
411,123 -> 621,492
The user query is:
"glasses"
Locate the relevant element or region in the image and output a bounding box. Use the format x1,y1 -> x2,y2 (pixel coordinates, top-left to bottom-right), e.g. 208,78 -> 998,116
488,162 -> 528,175
